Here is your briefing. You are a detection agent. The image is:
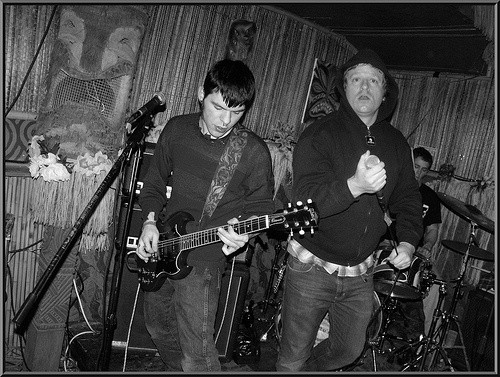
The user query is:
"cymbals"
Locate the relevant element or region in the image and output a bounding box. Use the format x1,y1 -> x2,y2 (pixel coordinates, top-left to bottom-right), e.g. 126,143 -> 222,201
440,239 -> 495,263
435,190 -> 495,236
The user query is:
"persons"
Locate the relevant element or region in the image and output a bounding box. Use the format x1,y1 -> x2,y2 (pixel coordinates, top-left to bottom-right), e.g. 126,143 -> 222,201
381,147 -> 445,343
136,59 -> 275,371
273,50 -> 424,372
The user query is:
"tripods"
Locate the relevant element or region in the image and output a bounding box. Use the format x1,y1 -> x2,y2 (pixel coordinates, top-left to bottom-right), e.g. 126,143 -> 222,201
350,173 -> 472,372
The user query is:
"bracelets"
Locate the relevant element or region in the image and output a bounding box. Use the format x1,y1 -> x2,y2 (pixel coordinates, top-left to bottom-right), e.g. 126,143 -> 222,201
143,220 -> 156,228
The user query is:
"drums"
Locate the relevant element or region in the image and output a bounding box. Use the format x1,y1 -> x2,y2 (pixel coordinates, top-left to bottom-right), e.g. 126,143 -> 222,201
374,279 -> 424,352
276,289 -> 384,370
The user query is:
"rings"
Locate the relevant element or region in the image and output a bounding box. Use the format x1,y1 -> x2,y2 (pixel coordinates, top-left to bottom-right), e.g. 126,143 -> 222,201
399,266 -> 402,268
145,244 -> 150,247
139,244 -> 141,247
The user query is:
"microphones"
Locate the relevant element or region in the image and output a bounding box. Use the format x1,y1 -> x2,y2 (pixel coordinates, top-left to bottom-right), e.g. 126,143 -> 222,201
126,91 -> 166,130
365,155 -> 386,213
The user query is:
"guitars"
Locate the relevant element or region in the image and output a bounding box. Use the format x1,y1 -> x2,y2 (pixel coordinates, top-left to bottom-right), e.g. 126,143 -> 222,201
136,197 -> 320,293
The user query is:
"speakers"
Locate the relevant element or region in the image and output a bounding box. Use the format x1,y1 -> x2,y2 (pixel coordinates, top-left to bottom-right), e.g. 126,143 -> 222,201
451,285 -> 495,371
